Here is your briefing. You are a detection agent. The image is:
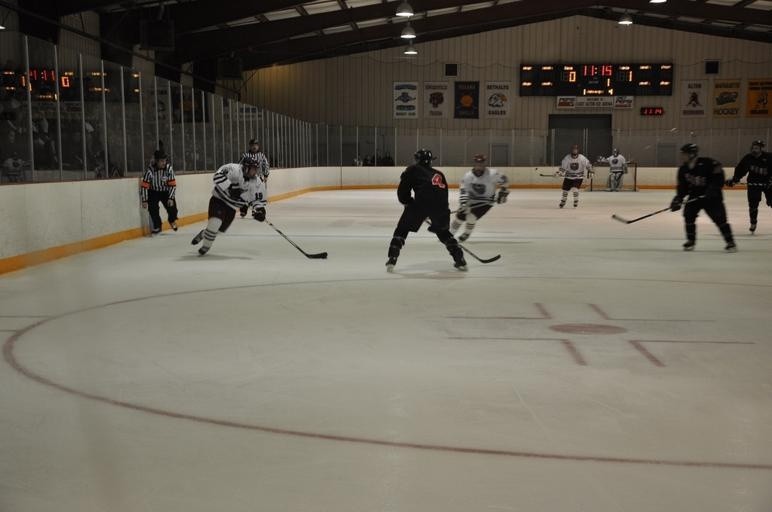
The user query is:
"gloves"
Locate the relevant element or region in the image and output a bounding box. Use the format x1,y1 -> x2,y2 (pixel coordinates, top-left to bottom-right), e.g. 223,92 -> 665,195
252,204 -> 265,221
726,177 -> 738,186
498,189 -> 508,203
671,196 -> 682,211
458,204 -> 468,214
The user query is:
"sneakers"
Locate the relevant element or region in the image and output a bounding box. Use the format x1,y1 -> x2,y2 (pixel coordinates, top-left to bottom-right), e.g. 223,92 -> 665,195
459,233 -> 469,240
455,258 -> 466,268
192,230 -> 210,254
750,224 -> 756,231
726,242 -> 734,249
386,256 -> 397,264
684,240 -> 694,246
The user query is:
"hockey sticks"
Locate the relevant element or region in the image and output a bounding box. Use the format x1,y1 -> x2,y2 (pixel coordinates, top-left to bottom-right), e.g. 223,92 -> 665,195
613,195 -> 704,224
252,208 -> 328,258
536,168 -> 587,177
425,220 -> 500,263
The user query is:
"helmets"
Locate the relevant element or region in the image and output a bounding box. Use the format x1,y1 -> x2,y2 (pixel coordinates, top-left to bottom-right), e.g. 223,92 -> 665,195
414,149 -> 433,164
473,154 -> 487,162
243,157 -> 259,174
680,144 -> 698,155
249,139 -> 259,145
751,140 -> 765,150
154,151 -> 167,160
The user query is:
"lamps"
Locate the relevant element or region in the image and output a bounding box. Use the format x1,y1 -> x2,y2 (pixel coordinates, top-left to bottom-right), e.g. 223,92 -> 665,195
394,0 -> 420,54
617,7 -> 634,25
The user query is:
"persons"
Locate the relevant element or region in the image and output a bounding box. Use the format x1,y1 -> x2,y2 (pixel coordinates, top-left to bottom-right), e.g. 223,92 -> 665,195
238,138 -> 270,216
191,156 -> 267,255
385,148 -> 467,268
140,151 -> 179,236
450,153 -> 510,241
554,144 -> 594,208
669,142 -> 736,250
596,147 -> 627,190
353,149 -> 395,166
725,139 -> 772,232
